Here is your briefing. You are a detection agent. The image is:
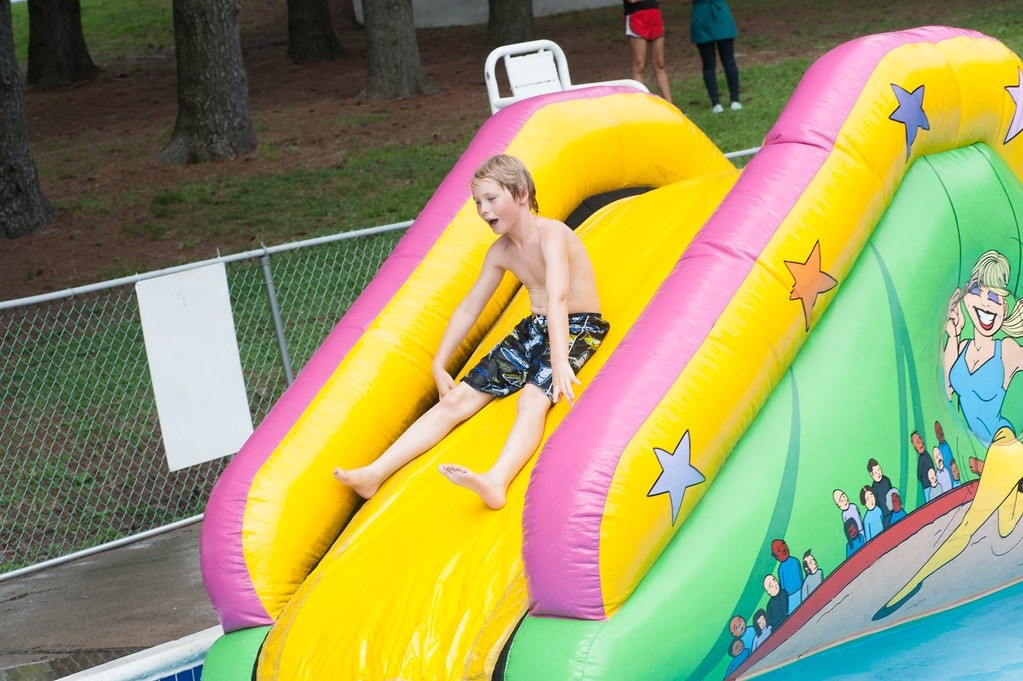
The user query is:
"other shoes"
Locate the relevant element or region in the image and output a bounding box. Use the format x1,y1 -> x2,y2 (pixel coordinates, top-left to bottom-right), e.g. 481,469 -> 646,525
714,103 -> 743,115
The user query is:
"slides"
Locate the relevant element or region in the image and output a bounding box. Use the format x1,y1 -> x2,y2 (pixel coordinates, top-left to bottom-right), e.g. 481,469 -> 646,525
251,171 -> 753,679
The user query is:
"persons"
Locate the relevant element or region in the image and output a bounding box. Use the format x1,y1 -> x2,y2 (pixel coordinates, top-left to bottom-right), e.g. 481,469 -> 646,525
686,1 -> 746,113
331,153 -> 615,509
620,0 -> 671,108
